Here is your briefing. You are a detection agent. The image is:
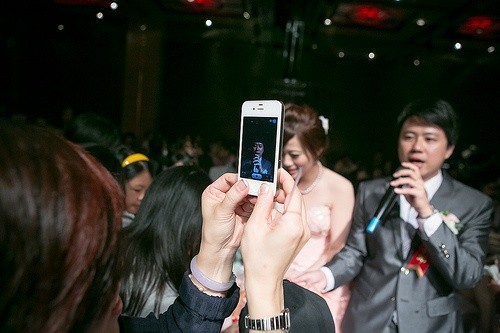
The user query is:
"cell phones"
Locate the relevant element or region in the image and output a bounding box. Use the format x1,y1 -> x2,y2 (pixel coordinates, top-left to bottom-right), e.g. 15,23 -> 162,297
238,100 -> 286,196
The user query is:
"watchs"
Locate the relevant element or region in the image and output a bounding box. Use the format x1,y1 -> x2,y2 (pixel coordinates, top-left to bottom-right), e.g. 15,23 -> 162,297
244,307 -> 291,333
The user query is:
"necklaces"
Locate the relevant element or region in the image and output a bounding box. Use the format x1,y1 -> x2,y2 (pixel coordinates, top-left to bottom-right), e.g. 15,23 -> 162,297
299,159 -> 323,193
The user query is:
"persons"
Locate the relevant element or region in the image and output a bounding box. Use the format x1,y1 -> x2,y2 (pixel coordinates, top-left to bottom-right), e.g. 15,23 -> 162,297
269,102 -> 356,333
0,127 -> 313,333
241,136 -> 272,175
294,95 -> 494,332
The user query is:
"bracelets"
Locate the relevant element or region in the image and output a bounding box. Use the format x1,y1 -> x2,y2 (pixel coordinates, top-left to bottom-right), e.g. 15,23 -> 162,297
190,254 -> 236,292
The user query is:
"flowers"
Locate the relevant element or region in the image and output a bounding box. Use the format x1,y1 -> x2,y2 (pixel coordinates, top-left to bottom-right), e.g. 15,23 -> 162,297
318,115 -> 329,136
440,211 -> 461,235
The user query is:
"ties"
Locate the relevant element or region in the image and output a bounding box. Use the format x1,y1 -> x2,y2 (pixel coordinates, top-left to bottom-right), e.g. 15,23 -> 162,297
255,165 -> 259,174
402,205 -> 419,260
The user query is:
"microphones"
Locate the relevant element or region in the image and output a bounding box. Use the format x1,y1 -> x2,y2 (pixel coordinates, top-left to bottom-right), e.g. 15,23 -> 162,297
254,154 -> 259,168
365,166 -> 410,234
